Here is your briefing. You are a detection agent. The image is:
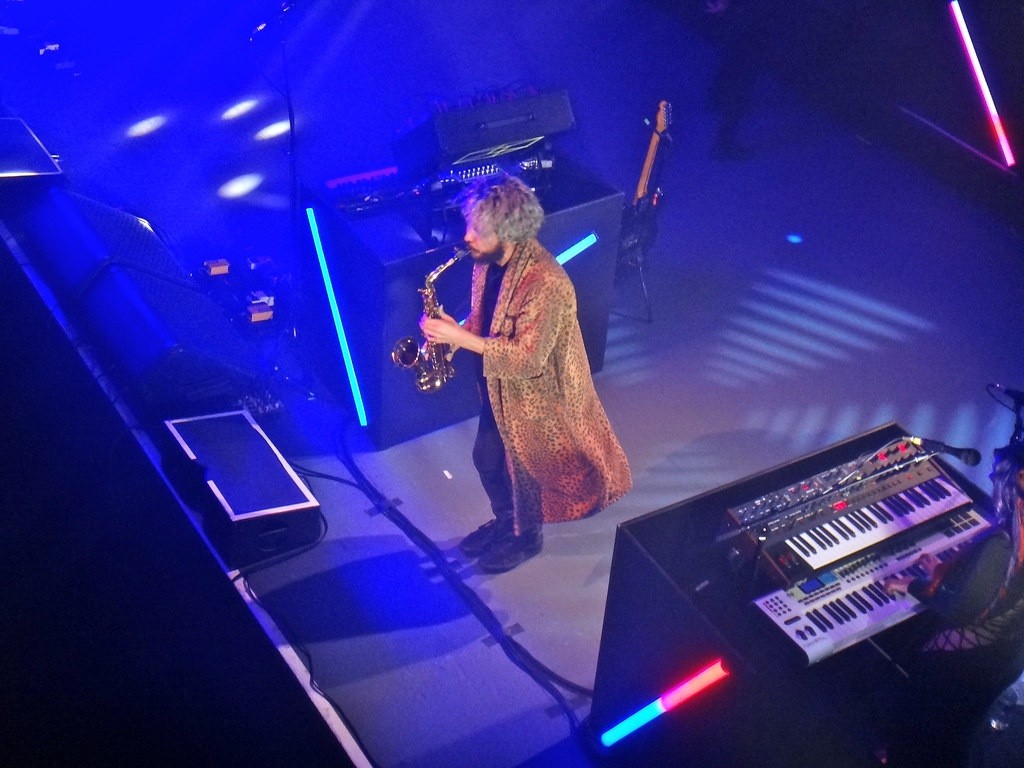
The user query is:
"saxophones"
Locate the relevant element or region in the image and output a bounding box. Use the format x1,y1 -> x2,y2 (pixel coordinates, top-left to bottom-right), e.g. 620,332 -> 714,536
391,245 -> 473,393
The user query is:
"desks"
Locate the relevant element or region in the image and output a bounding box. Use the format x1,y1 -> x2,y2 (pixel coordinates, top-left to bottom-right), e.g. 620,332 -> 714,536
316,152 -> 626,451
588,424 -> 996,767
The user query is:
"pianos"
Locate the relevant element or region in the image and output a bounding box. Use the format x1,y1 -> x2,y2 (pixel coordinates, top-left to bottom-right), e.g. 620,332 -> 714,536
715,438 -> 993,670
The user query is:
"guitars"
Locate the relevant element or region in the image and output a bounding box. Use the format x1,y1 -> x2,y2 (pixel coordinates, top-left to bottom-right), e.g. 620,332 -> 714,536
633,100 -> 672,207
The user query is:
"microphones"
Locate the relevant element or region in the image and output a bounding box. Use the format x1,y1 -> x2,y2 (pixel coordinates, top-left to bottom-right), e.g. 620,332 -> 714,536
252,4 -> 296,37
903,436 -> 982,467
992,383 -> 1024,405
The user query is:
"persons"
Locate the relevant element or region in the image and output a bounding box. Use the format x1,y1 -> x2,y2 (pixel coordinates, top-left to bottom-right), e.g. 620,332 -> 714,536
420,173 -> 633,571
877,445 -> 1024,768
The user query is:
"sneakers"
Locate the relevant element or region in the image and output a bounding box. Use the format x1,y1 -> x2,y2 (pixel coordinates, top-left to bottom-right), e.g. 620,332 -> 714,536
460,519 -> 514,556
478,532 -> 543,570
